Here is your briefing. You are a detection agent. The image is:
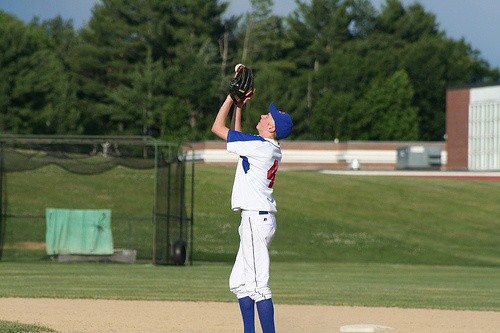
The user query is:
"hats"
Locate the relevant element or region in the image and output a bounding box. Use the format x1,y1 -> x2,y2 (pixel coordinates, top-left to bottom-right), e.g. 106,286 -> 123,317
269,103 -> 292,140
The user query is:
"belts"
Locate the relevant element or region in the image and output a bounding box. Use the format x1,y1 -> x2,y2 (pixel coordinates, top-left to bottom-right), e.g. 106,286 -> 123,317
259,210 -> 268,214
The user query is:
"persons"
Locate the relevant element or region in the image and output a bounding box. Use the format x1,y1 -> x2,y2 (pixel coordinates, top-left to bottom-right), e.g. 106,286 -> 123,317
211,66 -> 293,333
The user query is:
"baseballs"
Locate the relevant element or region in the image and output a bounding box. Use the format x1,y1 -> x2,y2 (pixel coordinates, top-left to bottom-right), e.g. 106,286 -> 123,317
234,63 -> 243,73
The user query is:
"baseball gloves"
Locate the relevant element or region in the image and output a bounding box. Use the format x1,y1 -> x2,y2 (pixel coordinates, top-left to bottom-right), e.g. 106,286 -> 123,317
228,66 -> 256,107
